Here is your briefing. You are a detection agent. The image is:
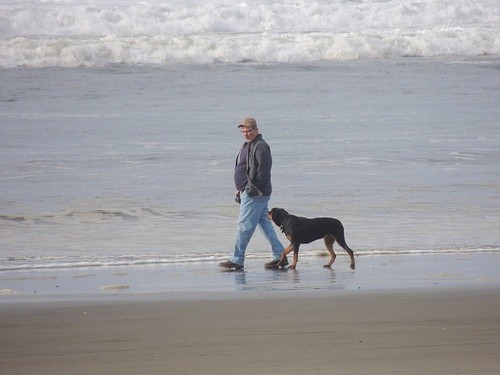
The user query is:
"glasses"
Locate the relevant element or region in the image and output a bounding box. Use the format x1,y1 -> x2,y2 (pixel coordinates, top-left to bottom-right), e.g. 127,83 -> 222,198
241,127 -> 255,133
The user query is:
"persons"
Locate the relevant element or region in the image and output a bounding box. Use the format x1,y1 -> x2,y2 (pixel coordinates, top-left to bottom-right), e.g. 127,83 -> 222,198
218,117 -> 289,268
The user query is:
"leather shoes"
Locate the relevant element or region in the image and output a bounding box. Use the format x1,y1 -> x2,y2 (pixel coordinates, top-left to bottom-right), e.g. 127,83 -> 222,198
265,257 -> 288,267
219,261 -> 244,268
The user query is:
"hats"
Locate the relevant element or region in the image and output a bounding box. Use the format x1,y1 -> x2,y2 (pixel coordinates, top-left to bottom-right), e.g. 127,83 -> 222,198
238,118 -> 257,128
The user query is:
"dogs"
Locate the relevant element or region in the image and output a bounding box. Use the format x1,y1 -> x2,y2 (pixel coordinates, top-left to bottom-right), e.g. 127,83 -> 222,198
268,207 -> 355,270
